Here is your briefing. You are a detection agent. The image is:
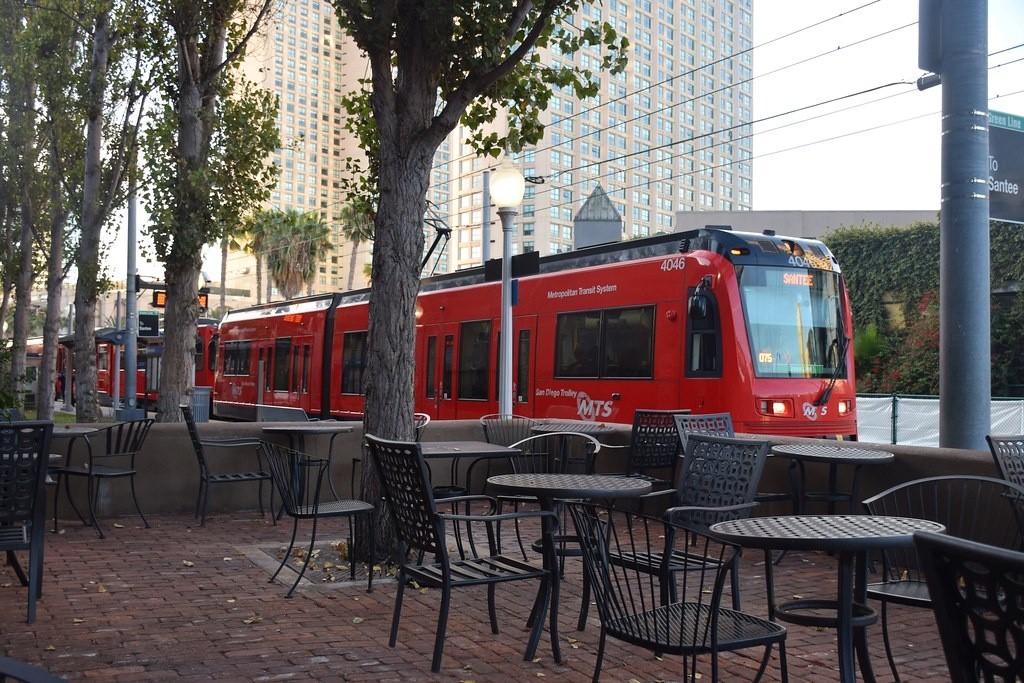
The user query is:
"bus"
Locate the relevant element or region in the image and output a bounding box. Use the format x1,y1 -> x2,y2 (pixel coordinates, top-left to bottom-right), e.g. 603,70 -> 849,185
1,318 -> 219,422
213,224 -> 858,441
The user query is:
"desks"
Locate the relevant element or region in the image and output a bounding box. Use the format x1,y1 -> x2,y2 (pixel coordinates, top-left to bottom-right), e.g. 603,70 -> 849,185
415,441 -> 522,566
21,427 -> 98,534
709,515 -> 946,683
531,424 -> 615,474
772,445 -> 894,574
262,425 -> 354,520
487,474 -> 652,662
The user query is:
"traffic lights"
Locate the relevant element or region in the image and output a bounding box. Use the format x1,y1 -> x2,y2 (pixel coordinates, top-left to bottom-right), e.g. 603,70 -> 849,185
151,290 -> 209,313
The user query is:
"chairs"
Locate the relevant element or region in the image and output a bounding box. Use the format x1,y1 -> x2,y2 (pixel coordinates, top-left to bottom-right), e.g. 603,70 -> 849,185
54,419 -> 156,539
258,440 -> 375,598
179,405 -> 275,527
364,433 -> 561,672
414,409 -> 1024,683
0,420 -> 54,624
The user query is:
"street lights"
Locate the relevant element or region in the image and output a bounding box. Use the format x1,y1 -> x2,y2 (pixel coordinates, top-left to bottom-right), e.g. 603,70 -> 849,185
489,151 -> 525,422
